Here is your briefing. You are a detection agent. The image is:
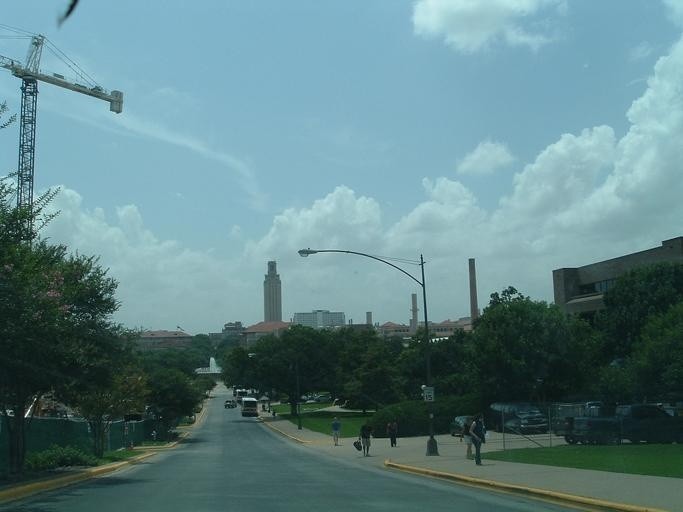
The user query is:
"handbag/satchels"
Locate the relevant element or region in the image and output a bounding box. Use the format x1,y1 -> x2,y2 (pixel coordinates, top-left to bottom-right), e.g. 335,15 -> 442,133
353,440 -> 362,450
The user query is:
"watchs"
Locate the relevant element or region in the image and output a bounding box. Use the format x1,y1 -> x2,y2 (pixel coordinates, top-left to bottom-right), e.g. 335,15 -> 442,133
389,420 -> 398,445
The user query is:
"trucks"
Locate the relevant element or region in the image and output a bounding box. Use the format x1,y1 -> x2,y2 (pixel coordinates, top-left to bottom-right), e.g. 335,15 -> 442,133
550,401 -> 587,439
490,402 -> 549,434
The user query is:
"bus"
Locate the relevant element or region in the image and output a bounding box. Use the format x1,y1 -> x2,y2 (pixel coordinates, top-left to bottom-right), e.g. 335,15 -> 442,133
235,389 -> 248,402
240,396 -> 258,418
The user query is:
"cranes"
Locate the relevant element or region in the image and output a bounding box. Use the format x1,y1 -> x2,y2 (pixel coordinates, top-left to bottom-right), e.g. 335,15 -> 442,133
0,19 -> 124,245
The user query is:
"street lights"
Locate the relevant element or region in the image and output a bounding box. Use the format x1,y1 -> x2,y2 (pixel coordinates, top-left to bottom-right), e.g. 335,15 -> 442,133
296,247 -> 441,457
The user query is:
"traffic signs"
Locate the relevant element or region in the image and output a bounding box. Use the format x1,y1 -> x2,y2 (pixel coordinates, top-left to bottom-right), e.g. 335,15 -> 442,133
423,387 -> 434,402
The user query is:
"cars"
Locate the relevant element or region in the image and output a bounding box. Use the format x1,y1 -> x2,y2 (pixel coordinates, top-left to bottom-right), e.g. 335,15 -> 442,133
448,415 -> 488,437
313,395 -> 332,402
223,399 -> 237,409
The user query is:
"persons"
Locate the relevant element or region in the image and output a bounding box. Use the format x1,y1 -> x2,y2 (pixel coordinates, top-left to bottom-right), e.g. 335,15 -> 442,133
330,416 -> 340,445
358,420 -> 375,456
460,415 -> 475,459
424,433 -> 439,456
468,414 -> 486,465
386,421 -> 395,444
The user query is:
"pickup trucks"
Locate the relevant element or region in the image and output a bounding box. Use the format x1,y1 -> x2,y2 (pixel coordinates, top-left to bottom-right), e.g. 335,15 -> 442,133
563,403 -> 683,446
